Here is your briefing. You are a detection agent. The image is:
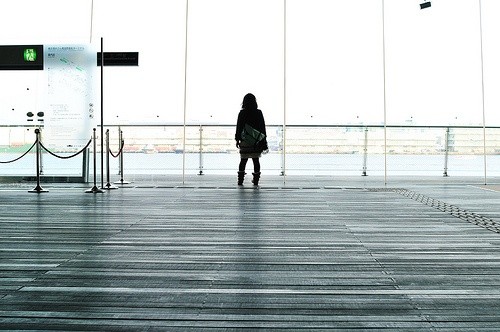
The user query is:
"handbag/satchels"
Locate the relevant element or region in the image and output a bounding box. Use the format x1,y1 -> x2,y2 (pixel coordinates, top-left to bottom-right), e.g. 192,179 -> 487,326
241,124 -> 265,146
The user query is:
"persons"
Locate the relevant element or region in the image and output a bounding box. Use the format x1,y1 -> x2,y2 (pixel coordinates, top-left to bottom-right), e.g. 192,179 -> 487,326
235,93 -> 268,186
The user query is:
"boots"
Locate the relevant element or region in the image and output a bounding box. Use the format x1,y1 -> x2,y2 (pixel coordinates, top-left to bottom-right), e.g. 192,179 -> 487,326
252,172 -> 260,185
238,171 -> 245,185
